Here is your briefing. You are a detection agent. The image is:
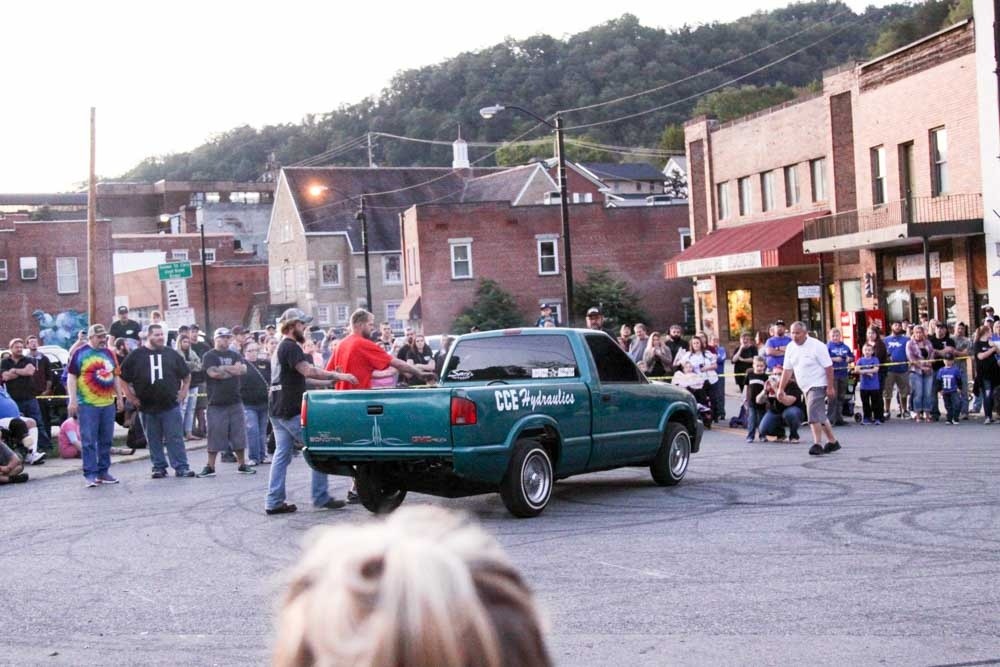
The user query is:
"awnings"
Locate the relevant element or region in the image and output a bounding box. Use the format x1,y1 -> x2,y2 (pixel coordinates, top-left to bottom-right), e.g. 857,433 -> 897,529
664,209 -> 833,280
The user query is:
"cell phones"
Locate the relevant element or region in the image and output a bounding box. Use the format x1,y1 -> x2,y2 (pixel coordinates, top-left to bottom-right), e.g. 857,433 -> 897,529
334,329 -> 343,335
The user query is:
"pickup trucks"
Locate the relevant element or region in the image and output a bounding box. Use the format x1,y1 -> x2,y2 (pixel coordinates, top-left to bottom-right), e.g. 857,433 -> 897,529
301,328 -> 704,515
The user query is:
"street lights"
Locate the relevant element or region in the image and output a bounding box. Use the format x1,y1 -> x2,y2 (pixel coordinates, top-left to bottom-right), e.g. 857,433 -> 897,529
306,183 -> 372,312
479,104 -> 574,327
160,213 -> 210,340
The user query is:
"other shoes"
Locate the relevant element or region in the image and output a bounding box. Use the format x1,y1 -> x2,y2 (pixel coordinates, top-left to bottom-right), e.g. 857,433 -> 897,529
152,468 -> 167,478
9,472 -> 28,483
220,452 -> 238,463
322,498 -> 346,509
175,465 -> 195,477
823,440 -> 841,453
345,491 -> 360,503
31,452 -> 46,464
745,411 -> 1000,443
266,503 -> 296,514
809,444 -> 824,455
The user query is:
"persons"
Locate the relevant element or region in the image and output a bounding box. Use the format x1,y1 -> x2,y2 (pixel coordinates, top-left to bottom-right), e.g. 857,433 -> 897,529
534,304 -> 559,327
774,320 -> 841,455
0,305 -> 460,489
261,309 -> 357,512
582,309 -> 1000,456
471,326 -> 479,332
324,305 -> 436,505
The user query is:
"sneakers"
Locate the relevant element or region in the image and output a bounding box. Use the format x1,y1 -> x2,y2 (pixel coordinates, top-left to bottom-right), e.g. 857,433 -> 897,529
95,473 -> 119,484
196,467 -> 217,478
85,479 -> 97,488
236,464 -> 256,474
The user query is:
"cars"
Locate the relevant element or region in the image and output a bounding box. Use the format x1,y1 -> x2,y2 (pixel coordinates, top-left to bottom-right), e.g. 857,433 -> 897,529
0,345 -> 69,405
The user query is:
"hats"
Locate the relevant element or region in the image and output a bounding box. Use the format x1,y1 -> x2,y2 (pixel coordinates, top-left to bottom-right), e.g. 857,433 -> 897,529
586,307 -> 602,316
214,327 -> 233,338
232,324 -> 249,335
279,308 -> 312,324
539,304 -> 549,310
118,306 -> 129,314
775,319 -> 786,325
88,324 -> 108,338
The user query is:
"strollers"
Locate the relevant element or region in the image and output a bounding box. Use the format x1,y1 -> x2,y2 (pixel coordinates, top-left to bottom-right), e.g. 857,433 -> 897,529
825,369 -> 862,423
